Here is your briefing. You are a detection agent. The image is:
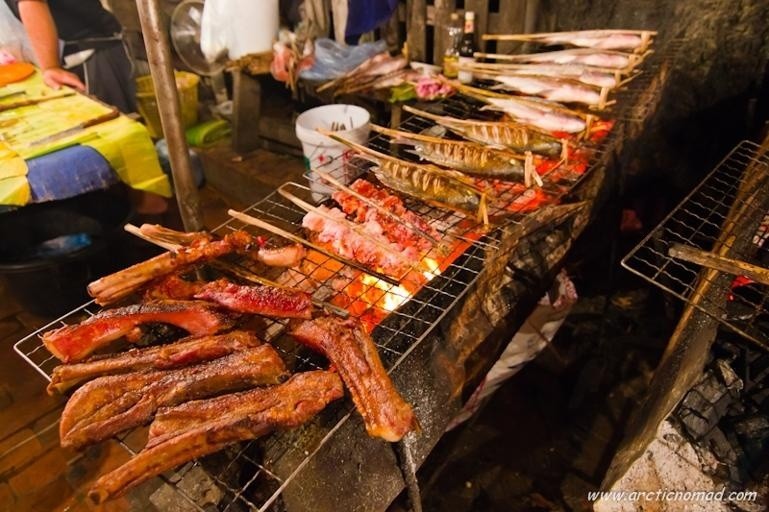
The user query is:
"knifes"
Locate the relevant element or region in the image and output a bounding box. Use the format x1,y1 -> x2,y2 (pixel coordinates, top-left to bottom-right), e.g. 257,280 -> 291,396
27,108 -> 121,147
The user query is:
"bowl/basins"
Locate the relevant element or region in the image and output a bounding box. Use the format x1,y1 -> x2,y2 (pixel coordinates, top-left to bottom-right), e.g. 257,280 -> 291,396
0,209 -> 103,318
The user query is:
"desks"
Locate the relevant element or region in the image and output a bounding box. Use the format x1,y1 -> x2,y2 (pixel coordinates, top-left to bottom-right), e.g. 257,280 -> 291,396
0,49 -> 176,215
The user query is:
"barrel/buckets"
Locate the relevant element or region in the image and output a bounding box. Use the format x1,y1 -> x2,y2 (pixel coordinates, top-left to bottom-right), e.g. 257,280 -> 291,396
135,69 -> 200,136
294,103 -> 372,205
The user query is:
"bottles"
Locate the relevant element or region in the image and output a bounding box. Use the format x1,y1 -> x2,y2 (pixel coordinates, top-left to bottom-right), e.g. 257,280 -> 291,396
444,13 -> 463,79
458,11 -> 481,88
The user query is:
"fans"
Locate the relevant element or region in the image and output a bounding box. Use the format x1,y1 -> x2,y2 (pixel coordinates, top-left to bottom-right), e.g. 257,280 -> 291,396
170,0 -> 232,105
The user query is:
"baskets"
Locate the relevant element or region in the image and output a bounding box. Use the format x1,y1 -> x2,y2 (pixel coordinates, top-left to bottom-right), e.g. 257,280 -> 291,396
133,72 -> 202,143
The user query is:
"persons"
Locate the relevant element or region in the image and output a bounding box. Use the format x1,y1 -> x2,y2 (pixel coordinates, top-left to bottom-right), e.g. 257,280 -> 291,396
3,0 -> 170,218
